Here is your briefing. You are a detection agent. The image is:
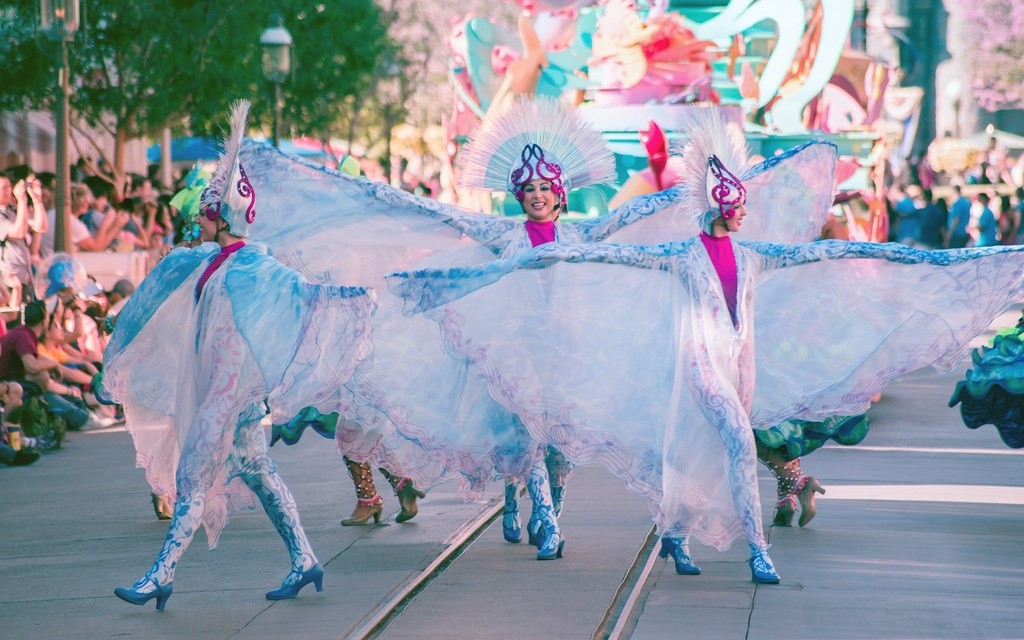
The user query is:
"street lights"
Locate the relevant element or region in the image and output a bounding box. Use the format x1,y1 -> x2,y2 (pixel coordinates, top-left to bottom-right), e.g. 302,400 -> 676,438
41,0 -> 82,250
259,15 -> 292,147
373,62 -> 401,185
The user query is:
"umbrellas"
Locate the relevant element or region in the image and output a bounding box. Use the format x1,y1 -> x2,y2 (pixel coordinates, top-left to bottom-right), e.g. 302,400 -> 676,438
148,136 -> 325,163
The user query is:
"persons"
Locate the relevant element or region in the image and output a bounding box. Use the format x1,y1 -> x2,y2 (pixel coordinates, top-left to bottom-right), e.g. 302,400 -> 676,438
0,154 -> 195,468
241,95 -> 1023,584
96,98 -> 377,611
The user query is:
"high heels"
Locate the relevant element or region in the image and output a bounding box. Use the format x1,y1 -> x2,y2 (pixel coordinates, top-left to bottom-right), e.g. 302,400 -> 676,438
746,558 -> 781,583
114,581 -> 173,611
537,530 -> 566,559
773,501 -> 795,527
395,478 -> 425,522
535,524 -> 546,550
341,498 -> 383,526
152,491 -> 174,520
658,531 -> 701,575
266,562 -> 324,600
527,519 -> 538,545
797,476 -> 825,527
502,521 -> 524,543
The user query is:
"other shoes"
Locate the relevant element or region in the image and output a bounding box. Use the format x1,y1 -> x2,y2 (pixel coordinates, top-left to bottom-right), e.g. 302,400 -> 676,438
49,416 -> 67,450
13,451 -> 41,465
79,413 -> 114,431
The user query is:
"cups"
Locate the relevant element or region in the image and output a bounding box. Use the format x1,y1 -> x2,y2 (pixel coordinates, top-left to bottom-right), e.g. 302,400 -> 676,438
8,426 -> 22,452
